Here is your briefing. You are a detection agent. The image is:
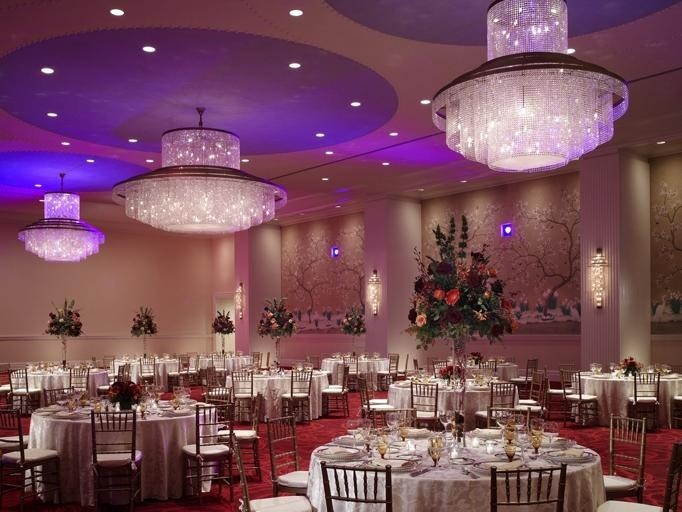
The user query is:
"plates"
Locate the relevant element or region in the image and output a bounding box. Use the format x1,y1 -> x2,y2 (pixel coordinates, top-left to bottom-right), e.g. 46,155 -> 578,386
34,385 -> 208,418
316,410 -> 594,478
394,367 -> 509,391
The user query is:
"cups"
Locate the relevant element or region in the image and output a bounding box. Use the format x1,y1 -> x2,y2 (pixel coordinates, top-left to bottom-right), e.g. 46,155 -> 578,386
124,352 -> 169,364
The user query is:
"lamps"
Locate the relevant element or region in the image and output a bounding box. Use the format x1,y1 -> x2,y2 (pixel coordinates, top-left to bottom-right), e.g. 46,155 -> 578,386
589,247 -> 607,310
234,280 -> 244,320
107,104 -> 289,240
429,1 -> 632,177
17,170 -> 109,263
368,268 -> 382,317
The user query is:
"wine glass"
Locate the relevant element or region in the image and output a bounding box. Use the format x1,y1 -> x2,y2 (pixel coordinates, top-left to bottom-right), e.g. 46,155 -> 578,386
330,352 -> 380,361
576,361 -> 680,379
245,361 -> 321,376
25,361 -> 59,374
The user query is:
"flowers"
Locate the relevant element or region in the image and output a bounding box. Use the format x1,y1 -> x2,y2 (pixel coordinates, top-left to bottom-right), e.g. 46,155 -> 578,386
342,303 -> 365,336
402,211 -> 522,377
212,309 -> 235,337
257,298 -> 297,341
43,296 -> 87,342
130,305 -> 160,339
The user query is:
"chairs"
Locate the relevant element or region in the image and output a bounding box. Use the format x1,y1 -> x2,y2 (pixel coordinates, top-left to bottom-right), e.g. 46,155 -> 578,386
1,351 -> 401,512
308,354 -> 682,512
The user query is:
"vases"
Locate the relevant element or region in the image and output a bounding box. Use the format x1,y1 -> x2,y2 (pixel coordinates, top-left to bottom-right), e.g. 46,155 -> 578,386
275,339 -> 281,365
352,335 -> 356,357
448,334 -> 469,379
220,335 -> 227,357
142,336 -> 148,360
62,338 -> 68,368
